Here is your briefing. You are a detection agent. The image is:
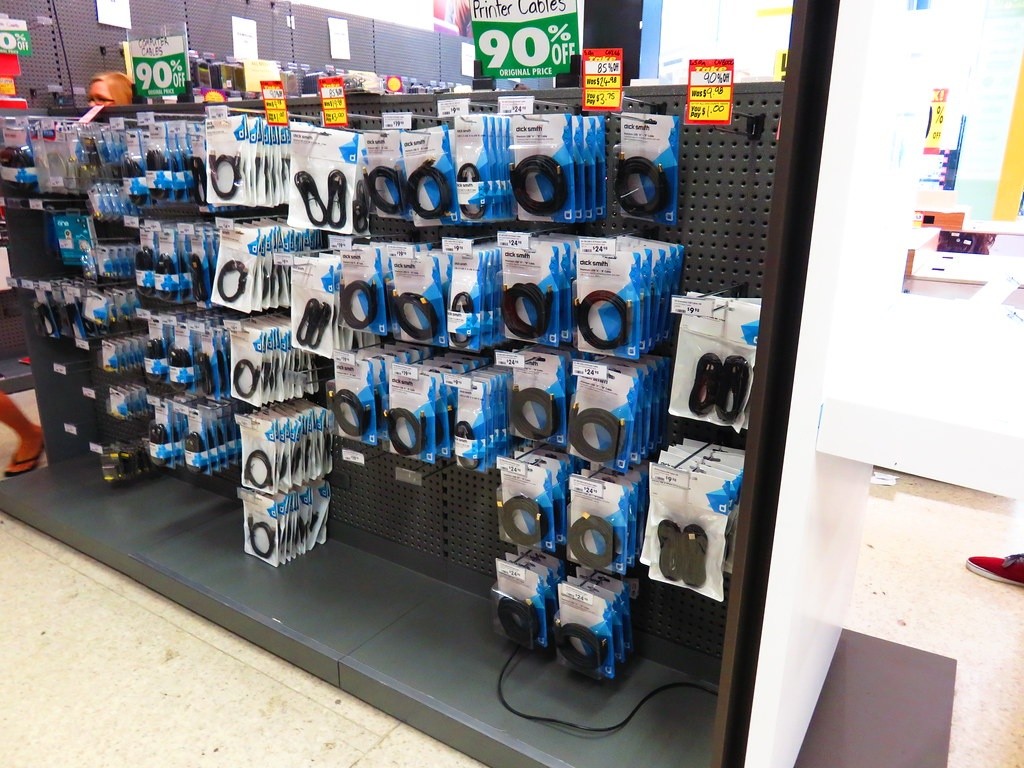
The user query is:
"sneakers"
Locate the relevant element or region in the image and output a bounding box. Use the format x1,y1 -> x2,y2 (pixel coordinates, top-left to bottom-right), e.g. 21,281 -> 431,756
966,553 -> 1024,587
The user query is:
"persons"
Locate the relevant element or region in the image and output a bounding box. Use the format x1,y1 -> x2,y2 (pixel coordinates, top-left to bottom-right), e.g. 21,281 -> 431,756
86,70 -> 134,112
0,392 -> 47,477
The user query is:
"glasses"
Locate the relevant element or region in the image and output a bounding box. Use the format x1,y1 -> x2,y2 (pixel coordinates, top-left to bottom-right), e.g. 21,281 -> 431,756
87,98 -> 113,105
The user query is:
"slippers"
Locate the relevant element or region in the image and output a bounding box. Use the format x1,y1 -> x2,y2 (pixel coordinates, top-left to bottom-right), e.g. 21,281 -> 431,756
5,443 -> 44,477
